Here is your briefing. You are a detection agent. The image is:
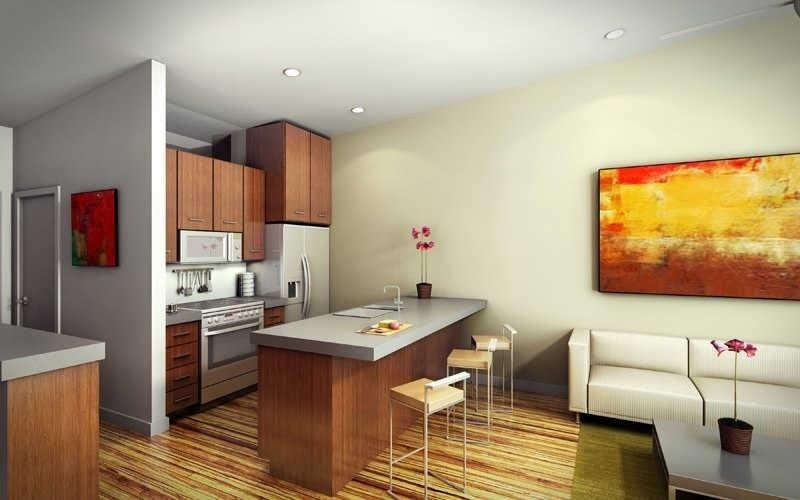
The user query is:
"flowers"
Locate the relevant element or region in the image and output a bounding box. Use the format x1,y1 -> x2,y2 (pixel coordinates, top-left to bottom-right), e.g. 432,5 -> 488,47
711,338 -> 758,423
410,223 -> 435,284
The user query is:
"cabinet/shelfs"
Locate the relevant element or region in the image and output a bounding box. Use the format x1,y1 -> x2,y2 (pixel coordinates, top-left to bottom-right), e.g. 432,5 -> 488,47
176,146 -> 244,233
264,306 -> 285,329
166,321 -> 200,424
242,164 -> 266,262
166,143 -> 179,264
246,119 -> 332,227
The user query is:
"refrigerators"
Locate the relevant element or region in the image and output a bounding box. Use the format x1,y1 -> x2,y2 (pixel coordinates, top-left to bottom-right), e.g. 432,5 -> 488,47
246,221 -> 330,326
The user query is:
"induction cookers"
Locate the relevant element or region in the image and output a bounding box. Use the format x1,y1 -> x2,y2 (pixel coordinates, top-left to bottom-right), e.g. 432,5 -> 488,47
176,296 -> 264,315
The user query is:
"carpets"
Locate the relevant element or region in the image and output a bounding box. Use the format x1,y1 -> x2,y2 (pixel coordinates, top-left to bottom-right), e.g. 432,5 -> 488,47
570,421 -> 725,500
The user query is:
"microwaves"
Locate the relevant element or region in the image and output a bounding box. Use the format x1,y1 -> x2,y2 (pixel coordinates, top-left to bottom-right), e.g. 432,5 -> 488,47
178,228 -> 243,263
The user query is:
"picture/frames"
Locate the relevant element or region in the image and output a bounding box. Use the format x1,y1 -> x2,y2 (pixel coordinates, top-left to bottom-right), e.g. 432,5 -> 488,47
598,154 -> 800,300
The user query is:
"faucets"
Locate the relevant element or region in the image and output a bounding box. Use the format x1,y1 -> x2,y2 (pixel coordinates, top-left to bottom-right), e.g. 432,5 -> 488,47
382,283 -> 404,312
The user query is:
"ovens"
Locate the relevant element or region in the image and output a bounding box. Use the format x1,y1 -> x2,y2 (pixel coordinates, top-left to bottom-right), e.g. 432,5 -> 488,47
197,304 -> 264,405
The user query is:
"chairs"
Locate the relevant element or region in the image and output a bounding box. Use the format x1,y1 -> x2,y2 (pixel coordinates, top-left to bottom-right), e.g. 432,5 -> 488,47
445,337 -> 497,442
386,371 -> 477,500
471,323 -> 518,414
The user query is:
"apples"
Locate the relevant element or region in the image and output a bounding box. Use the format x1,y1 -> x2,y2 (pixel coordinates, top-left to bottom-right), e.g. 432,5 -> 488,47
389,322 -> 400,329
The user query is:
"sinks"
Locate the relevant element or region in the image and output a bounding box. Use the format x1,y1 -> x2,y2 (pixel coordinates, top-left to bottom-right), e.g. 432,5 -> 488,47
361,303 -> 405,310
334,307 -> 390,319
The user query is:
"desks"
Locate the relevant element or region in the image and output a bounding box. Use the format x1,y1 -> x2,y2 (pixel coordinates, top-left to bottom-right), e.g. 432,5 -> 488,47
0,323 -> 106,500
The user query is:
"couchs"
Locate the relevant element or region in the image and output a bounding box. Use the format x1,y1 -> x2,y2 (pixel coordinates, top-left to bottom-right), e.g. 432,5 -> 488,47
568,326 -> 800,442
